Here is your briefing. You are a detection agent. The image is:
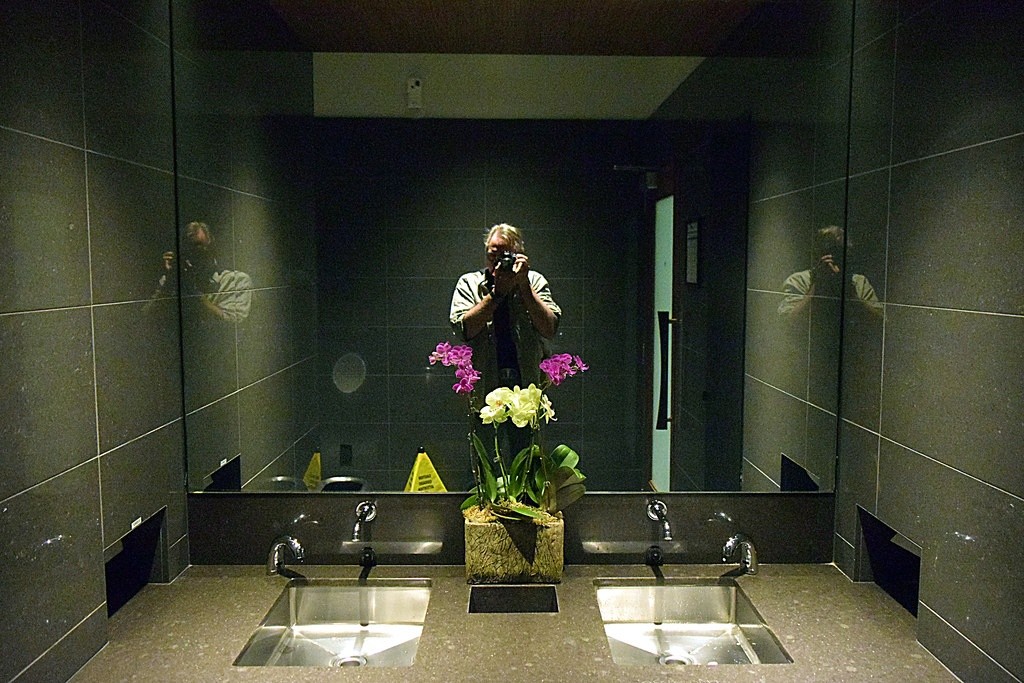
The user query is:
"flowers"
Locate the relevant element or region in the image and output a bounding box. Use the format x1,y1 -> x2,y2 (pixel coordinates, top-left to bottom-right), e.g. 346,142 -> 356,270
428,343 -> 589,523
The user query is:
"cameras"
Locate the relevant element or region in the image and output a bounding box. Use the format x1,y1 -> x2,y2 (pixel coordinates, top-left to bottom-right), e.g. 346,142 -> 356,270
494,251 -> 520,273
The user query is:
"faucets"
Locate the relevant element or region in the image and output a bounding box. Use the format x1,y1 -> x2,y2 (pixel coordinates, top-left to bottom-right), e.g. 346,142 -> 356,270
289,510 -> 326,530
646,500 -> 673,542
266,535 -> 306,577
721,533 -> 758,576
351,500 -> 377,542
701,510 -> 749,538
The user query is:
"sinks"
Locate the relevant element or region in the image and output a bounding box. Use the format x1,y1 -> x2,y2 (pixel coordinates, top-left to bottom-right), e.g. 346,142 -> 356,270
590,577 -> 793,666
231,578 -> 435,669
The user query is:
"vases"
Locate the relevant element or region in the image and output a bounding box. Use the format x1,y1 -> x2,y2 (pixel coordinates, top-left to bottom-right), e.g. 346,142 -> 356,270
465,511 -> 564,584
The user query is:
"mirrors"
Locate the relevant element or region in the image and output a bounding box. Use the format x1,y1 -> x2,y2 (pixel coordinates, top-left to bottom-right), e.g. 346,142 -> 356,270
171,0 -> 855,494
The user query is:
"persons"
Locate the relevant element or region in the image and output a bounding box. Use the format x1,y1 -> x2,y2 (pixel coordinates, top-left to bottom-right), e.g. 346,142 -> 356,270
774,226 -> 885,347
450,223 -> 562,477
144,219 -> 253,385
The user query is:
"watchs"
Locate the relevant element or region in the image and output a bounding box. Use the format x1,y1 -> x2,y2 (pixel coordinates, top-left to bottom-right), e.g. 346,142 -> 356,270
490,290 -> 502,304
